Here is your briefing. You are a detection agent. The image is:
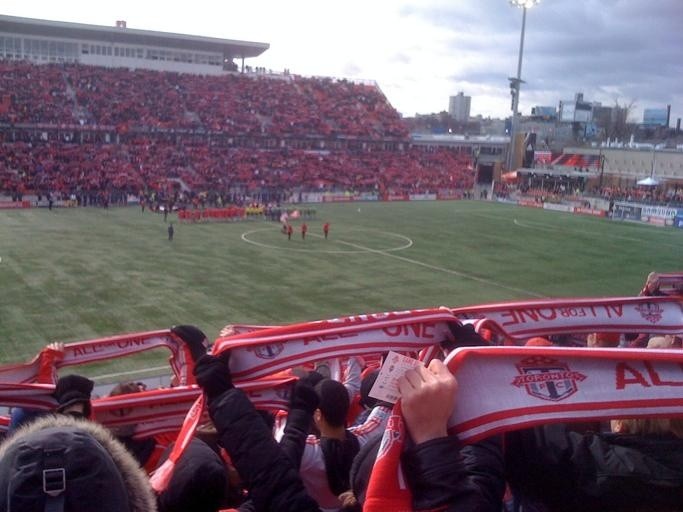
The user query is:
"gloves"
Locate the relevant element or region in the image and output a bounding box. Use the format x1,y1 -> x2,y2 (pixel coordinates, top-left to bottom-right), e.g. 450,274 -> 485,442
168,323 -> 210,360
439,317 -> 476,350
52,372 -> 95,417
290,371 -> 322,416
188,349 -> 235,399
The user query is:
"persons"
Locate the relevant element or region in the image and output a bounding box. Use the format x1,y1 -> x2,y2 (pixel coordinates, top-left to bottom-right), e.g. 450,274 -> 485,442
479,174 -> 683,218
282,218 -> 288,233
2,272 -> 683,512
286,224 -> 293,240
167,223 -> 174,241
301,223 -> 307,240
323,222 -> 330,239
1,52 -> 479,225
